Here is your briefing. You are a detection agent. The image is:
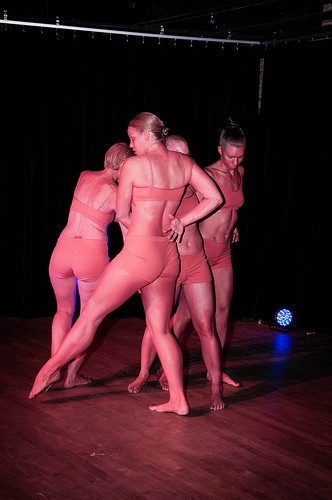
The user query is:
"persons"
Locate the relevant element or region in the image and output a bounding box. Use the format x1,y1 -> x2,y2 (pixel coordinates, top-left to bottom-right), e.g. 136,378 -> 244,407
159,118 -> 245,390
128,134 -> 224,410
29,112 -> 223,415
41,143 -> 137,393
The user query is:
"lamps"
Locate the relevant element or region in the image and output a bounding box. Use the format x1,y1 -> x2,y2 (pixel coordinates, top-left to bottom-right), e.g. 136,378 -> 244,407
271,305 -> 296,332
